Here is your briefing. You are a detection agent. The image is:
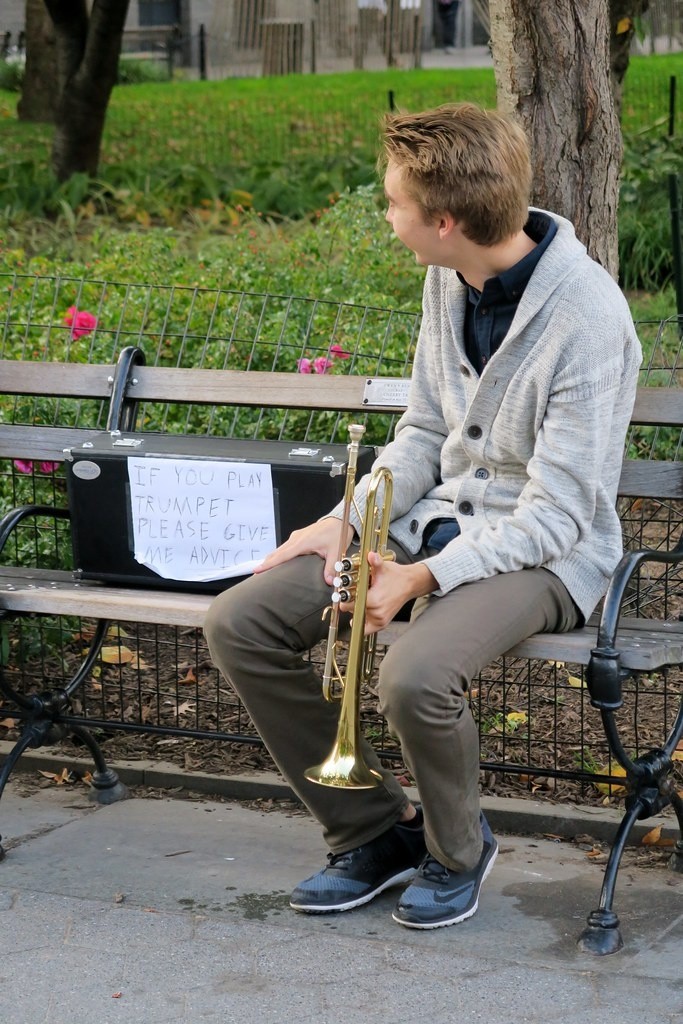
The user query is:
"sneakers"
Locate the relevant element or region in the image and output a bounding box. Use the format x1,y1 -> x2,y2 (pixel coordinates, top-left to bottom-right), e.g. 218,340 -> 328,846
289,808 -> 426,912
391,810 -> 499,929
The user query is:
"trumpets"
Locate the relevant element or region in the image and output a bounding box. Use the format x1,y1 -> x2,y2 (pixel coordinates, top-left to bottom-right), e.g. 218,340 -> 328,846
305,421 -> 400,790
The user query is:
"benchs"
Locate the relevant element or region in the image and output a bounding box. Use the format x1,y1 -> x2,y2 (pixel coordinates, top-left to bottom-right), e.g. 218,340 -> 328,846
0,345 -> 683,955
15,22 -> 183,80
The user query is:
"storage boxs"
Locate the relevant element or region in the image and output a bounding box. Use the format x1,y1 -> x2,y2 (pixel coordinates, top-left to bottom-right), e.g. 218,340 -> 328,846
63,431 -> 379,595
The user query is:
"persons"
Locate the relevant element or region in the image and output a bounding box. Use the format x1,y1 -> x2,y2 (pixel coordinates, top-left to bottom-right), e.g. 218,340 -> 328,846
202,101 -> 645,929
